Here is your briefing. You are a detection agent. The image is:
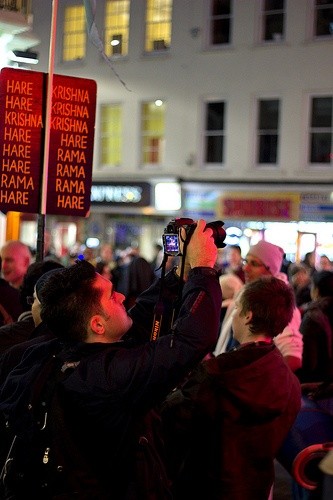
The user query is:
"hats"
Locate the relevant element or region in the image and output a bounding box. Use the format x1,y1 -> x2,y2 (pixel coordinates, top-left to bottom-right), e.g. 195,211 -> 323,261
248,241 -> 284,279
34,268 -> 61,305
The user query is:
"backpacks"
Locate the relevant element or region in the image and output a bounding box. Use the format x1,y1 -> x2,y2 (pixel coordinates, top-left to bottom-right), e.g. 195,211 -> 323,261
0,341 -> 101,500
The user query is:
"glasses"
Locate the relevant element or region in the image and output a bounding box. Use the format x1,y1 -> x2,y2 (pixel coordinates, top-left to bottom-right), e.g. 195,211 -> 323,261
26,296 -> 38,305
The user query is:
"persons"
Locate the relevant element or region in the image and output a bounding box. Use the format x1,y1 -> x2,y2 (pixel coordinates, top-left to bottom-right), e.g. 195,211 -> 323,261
0,234 -> 333,391
153,277 -> 301,500
0,218 -> 223,498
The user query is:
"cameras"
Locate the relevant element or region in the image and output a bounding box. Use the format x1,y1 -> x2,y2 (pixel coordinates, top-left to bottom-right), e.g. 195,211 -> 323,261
162,217 -> 227,256
